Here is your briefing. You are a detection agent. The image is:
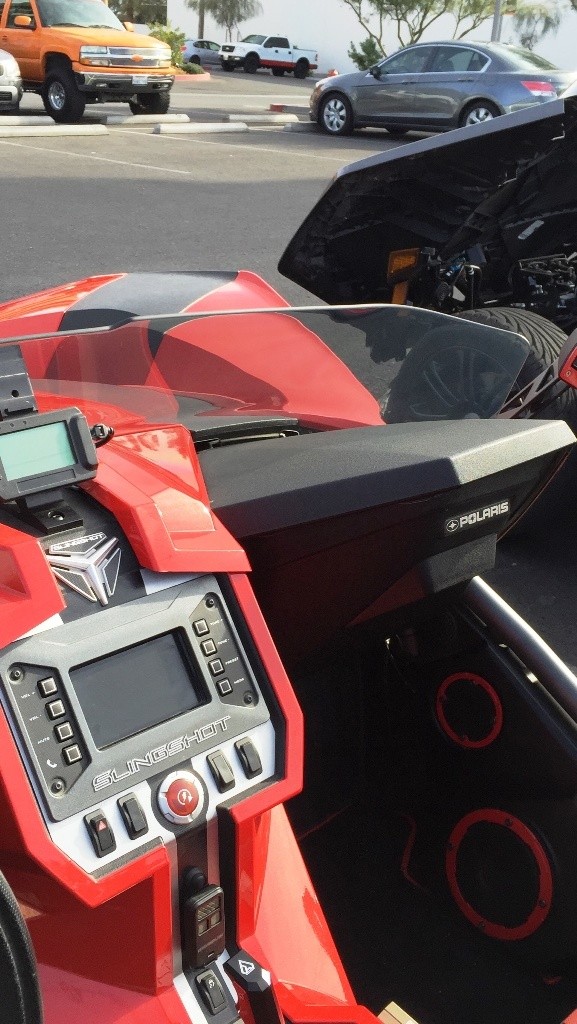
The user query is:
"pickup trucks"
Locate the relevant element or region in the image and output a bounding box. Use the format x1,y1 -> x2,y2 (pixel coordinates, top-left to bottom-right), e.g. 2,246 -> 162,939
216,33 -> 324,78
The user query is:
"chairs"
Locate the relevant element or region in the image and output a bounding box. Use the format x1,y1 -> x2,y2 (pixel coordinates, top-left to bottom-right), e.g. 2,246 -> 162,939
434,60 -> 454,72
467,60 -> 483,71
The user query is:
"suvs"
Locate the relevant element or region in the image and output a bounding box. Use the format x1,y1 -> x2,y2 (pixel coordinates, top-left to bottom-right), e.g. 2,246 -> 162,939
0,2 -> 175,124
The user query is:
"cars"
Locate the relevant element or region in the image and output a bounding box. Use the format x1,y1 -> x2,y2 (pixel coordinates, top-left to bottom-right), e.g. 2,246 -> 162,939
0,50 -> 27,110
309,41 -> 577,139
179,39 -> 225,64
